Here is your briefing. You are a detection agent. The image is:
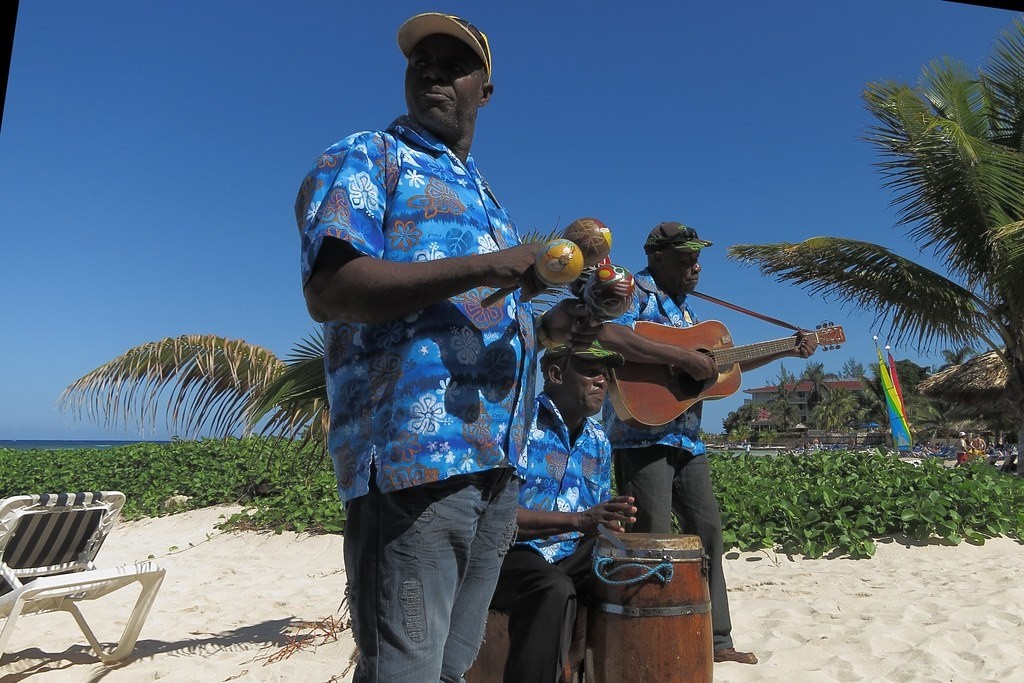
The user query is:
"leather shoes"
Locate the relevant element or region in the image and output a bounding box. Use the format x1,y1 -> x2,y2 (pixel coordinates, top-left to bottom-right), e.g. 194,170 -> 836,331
714,647 -> 758,664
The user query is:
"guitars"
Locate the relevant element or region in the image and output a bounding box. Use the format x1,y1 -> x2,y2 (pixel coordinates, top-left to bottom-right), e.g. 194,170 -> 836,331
606,319 -> 846,431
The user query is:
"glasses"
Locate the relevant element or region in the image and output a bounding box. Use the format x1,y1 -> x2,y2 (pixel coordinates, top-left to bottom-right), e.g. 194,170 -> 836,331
963,435 -> 966,436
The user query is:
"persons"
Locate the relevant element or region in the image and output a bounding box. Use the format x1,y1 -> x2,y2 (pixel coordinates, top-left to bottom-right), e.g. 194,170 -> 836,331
797,436 -> 843,451
934,444 -> 948,454
746,442 -> 751,453
923,441 -> 932,453
953,431 -> 1019,467
293,12 -> 819,683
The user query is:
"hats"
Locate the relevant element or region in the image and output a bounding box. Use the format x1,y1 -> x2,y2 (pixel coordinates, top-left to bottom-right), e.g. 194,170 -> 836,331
396,13 -> 491,84
540,338 -> 625,374
976,434 -> 980,437
748,442 -> 751,444
959,431 -> 966,437
644,222 -> 714,256
814,436 -> 817,439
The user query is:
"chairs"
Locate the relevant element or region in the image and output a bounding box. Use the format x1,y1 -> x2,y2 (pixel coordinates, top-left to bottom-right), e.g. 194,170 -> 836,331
0,491 -> 167,663
798,443 -> 1019,471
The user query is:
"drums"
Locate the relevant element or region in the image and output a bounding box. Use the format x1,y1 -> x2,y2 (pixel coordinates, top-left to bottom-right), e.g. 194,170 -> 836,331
581,533 -> 716,683
